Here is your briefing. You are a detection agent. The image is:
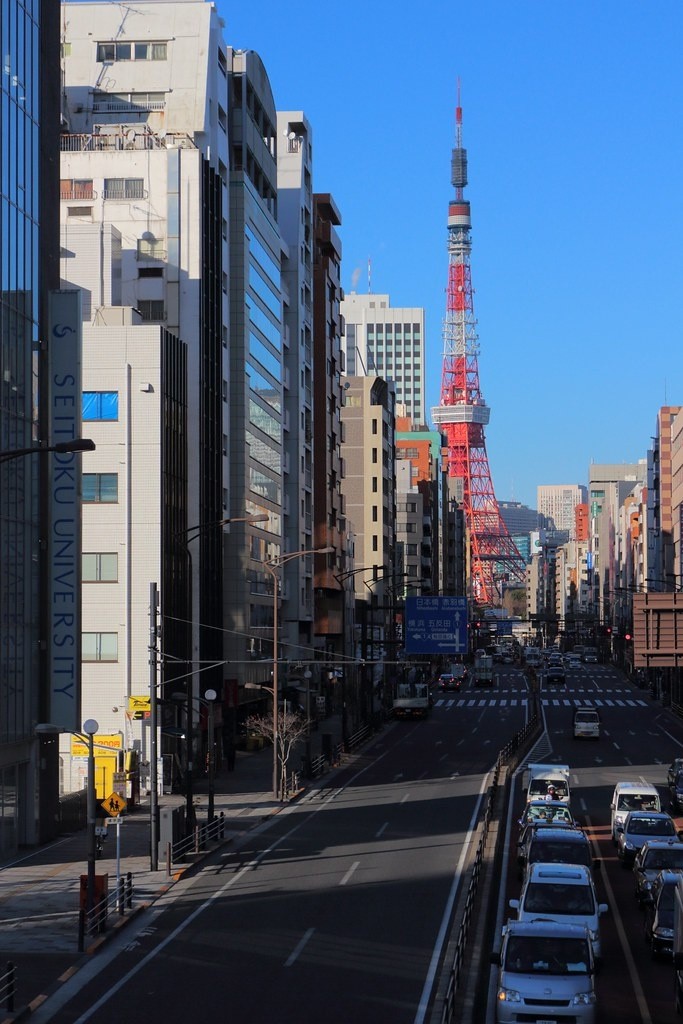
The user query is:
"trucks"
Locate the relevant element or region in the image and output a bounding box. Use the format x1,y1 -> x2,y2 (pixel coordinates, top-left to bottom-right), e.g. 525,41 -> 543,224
526,765 -> 570,801
451,663 -> 468,678
393,683 -> 431,719
574,708 -> 600,737
474,655 -> 494,686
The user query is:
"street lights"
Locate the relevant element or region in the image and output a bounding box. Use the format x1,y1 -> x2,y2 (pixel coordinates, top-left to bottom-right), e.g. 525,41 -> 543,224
576,574 -> 683,703
172,690 -> 217,836
245,547 -> 336,797
36,719 -> 99,935
148,515 -> 268,868
365,572 -> 433,738
334,565 -> 391,741
0,440 -> 98,461
76,739 -> 119,913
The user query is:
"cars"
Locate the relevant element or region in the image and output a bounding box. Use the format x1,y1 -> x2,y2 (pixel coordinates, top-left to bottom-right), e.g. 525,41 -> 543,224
522,828 -> 590,862
495,643 -> 599,683
525,801 -> 574,828
645,868 -> 683,945
632,840 -> 683,894
614,812 -> 677,865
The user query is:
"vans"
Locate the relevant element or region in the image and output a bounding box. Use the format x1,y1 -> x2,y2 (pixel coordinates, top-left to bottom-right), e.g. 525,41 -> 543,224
495,922 -> 594,1024
516,862 -> 599,957
438,674 -> 454,688
610,781 -> 661,842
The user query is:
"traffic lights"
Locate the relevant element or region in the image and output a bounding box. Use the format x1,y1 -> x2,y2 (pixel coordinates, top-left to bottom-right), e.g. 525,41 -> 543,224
599,626 -> 612,633
626,634 -> 630,647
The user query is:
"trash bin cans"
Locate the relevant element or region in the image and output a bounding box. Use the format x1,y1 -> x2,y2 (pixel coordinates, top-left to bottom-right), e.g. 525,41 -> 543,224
304,735 -> 324,778
81,872 -> 109,936
